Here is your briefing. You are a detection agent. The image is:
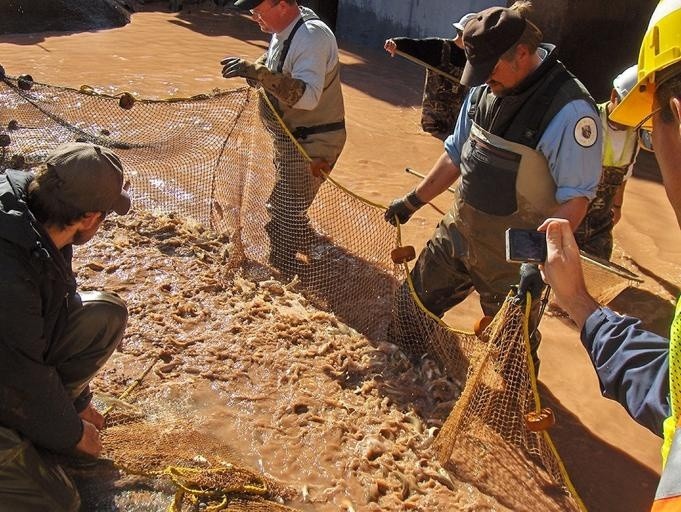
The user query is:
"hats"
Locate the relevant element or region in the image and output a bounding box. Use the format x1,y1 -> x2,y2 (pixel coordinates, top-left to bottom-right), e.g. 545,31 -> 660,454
47,142 -> 131,216
233,0 -> 263,10
452,7 -> 525,88
609,0 -> 681,130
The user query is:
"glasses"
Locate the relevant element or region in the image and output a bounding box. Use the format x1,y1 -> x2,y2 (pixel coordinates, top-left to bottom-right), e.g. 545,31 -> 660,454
634,106 -> 670,153
244,3 -> 278,15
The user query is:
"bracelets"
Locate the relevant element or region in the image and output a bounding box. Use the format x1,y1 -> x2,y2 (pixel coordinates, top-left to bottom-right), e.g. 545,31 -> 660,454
407,187 -> 428,210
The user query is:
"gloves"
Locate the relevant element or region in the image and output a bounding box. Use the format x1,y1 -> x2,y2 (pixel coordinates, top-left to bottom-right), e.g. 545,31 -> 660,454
220,55 -> 305,106
386,188 -> 425,226
511,263 -> 547,307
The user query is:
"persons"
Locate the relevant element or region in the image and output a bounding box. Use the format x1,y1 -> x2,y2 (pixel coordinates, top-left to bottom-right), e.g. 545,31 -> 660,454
570,62 -> 654,262
219,0 -> 346,275
374,2 -> 603,404
535,1 -> 680,512
383,10 -> 476,142
0,139 -> 138,472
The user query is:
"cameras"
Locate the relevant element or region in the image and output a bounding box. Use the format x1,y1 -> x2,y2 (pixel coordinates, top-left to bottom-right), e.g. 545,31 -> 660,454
504,227 -> 546,264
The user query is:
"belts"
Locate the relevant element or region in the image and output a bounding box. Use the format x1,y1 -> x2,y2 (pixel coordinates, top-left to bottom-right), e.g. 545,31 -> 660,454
291,120 -> 345,139
